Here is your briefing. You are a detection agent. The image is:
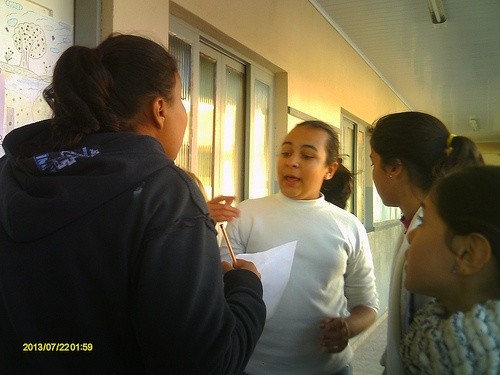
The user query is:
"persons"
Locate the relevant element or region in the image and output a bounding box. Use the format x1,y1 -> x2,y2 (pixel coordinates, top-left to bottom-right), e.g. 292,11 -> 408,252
400,162 -> 500,375
0,30 -> 267,374
206,115 -> 378,374
370,111 -> 488,375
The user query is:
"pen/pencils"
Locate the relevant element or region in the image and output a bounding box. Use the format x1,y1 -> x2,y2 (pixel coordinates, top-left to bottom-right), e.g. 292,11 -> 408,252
220,223 -> 237,268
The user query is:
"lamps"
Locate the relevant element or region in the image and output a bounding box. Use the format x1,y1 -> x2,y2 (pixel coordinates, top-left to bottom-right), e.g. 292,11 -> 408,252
426,1 -> 447,25
470,119 -> 480,132
